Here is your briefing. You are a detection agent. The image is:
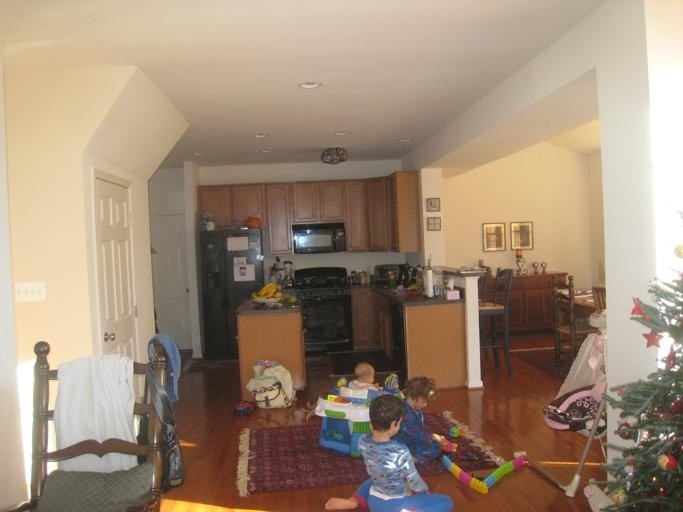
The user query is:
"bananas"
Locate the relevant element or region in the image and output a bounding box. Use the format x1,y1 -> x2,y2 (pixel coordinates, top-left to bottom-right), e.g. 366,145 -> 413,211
258,283 -> 277,299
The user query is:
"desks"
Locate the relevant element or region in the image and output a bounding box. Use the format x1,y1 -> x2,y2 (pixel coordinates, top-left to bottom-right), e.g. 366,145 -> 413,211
559,295 -> 597,327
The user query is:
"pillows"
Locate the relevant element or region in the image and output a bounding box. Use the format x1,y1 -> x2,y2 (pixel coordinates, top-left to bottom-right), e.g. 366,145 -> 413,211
34,462 -> 153,512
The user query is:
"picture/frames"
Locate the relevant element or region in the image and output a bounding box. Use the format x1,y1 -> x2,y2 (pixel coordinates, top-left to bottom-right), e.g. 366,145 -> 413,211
482,222 -> 506,251
426,198 -> 440,212
510,221 -> 533,250
428,217 -> 441,231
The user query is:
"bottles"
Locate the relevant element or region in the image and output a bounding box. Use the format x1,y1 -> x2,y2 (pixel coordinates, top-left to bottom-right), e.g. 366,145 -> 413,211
349,270 -> 370,285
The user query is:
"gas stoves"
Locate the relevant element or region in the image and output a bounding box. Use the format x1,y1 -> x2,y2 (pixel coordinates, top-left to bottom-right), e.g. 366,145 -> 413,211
299,282 -> 346,295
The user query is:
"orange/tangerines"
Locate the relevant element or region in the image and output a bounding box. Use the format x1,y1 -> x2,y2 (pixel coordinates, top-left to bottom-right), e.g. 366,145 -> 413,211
251,292 -> 257,299
275,291 -> 283,299
289,296 -> 296,304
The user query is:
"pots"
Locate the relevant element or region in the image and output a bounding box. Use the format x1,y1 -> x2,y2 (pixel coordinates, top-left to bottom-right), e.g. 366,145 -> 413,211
327,277 -> 344,282
302,277 -> 316,283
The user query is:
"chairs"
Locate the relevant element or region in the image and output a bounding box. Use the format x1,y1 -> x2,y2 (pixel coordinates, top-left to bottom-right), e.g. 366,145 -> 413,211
480,266 -> 490,367
11,334 -> 170,512
480,269 -> 514,375
593,287 -> 606,311
552,275 -> 598,366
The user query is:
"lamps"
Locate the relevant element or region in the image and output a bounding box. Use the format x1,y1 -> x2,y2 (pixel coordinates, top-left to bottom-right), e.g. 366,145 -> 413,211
515,248 -> 528,276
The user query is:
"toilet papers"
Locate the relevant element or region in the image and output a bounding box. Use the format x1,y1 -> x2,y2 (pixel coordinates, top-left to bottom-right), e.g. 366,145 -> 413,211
423,270 -> 434,298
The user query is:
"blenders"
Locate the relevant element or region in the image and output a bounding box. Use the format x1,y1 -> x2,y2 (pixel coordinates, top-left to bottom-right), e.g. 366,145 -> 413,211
283,261 -> 296,286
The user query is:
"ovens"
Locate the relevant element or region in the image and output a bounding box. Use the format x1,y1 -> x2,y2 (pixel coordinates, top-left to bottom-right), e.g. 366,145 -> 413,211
301,297 -> 352,352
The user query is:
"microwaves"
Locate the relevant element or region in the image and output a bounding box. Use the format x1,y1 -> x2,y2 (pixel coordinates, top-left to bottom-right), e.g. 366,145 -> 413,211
375,265 -> 408,280
295,230 -> 345,253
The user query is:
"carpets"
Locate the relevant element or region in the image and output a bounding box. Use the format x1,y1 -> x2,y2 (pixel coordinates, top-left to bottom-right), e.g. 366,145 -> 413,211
326,350 -> 401,377
509,348 -> 569,381
236,410 -> 506,497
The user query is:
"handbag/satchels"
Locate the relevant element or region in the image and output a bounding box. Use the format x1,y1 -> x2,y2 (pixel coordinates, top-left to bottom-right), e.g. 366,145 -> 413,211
134,415 -> 186,493
252,380 -> 296,409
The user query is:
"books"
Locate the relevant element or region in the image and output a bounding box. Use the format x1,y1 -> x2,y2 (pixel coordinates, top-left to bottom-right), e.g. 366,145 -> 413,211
561,289 -> 593,303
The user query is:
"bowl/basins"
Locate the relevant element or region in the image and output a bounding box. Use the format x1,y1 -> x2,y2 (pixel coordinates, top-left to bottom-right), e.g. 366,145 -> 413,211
252,298 -> 284,304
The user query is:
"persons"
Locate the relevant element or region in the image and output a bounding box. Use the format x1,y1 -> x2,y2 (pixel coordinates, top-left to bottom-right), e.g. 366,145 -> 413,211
325,396 -> 454,512
348,360 -> 379,391
389,376 -> 442,465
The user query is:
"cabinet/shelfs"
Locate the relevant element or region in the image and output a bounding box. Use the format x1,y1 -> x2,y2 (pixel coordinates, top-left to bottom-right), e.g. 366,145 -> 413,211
352,289 -> 375,351
479,271 -> 569,335
197,170 -> 421,257
372,290 -> 393,362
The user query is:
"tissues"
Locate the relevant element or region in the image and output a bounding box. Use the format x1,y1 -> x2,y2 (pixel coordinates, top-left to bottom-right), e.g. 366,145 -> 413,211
443,279 -> 460,300
